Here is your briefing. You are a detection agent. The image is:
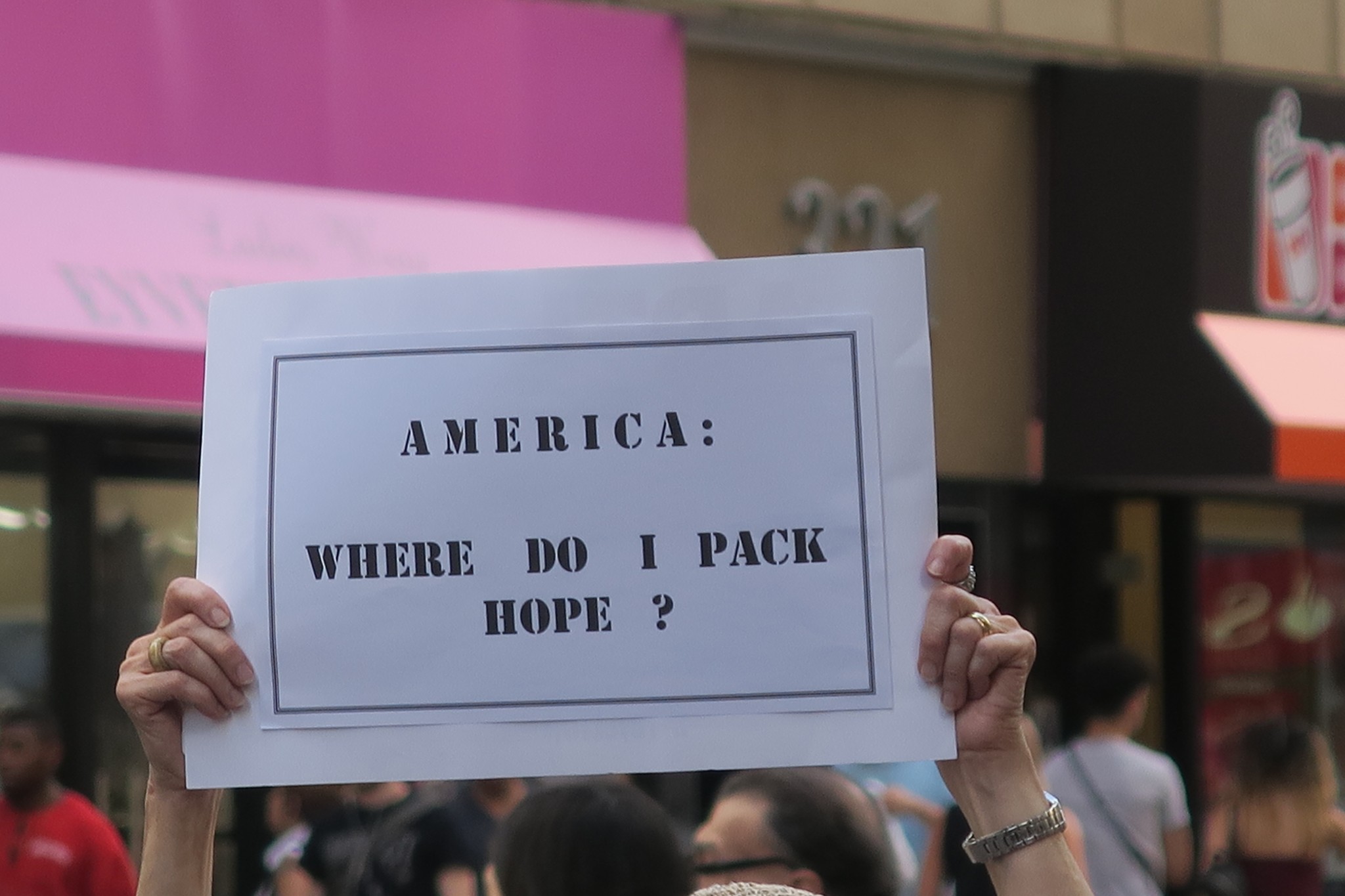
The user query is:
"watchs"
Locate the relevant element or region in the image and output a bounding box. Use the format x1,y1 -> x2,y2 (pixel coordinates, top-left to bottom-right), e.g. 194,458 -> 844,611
962,790 -> 1068,864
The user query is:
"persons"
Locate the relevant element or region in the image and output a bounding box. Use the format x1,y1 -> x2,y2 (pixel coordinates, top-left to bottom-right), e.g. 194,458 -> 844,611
0,532 -> 1345,896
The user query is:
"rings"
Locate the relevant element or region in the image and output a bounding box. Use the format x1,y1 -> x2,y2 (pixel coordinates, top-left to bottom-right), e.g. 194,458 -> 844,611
965,610 -> 992,638
939,565 -> 976,591
148,636 -> 170,672
